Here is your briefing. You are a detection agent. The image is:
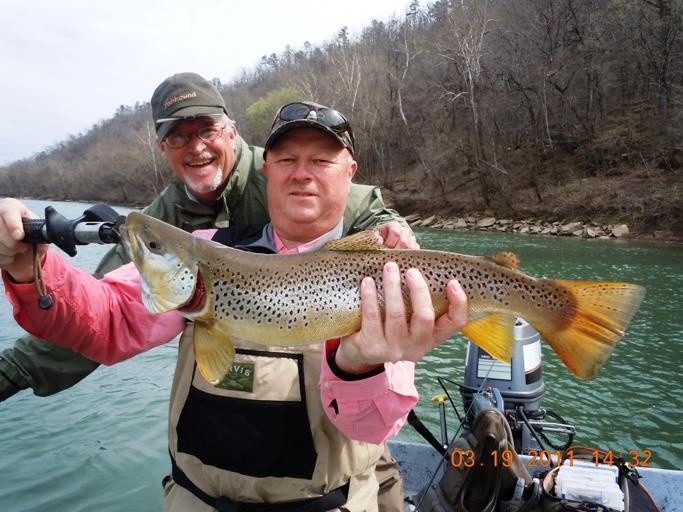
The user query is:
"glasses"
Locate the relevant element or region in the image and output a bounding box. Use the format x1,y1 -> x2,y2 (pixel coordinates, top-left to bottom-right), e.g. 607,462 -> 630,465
271,102 -> 354,149
165,124 -> 227,149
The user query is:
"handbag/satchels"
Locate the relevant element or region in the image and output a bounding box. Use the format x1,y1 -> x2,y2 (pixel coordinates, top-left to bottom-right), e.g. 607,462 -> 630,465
543,446 -> 660,512
416,410 -> 532,512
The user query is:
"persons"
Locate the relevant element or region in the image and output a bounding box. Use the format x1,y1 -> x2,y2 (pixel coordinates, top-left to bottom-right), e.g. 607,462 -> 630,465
0,101 -> 470,512
1,72 -> 420,511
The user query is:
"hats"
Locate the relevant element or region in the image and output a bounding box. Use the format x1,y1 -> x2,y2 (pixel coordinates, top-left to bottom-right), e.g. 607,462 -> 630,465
151,72 -> 228,143
263,101 -> 354,161
